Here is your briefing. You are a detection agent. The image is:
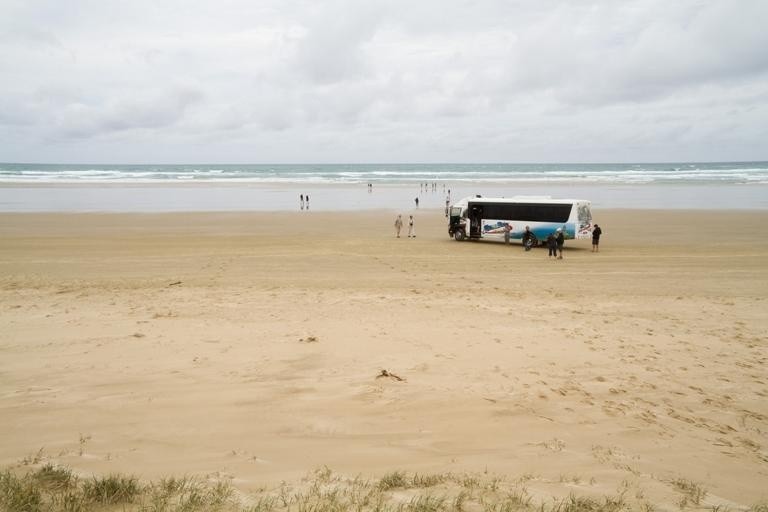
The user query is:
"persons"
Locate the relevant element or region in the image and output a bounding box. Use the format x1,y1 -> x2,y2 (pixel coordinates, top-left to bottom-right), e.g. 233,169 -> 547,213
547,233 -> 557,257
591,224 -> 602,252
522,226 -> 530,251
555,228 -> 564,259
408,215 -> 416,238
394,214 -> 403,238
504,223 -> 510,245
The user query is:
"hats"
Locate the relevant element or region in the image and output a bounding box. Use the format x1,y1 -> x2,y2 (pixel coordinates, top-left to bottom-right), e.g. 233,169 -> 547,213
556,228 -> 561,232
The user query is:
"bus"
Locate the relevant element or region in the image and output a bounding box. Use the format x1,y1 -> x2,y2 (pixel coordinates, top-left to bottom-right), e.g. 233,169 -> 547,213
446,193 -> 593,245
